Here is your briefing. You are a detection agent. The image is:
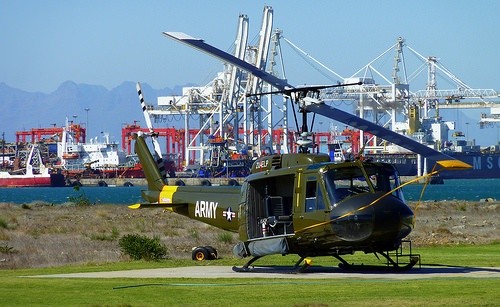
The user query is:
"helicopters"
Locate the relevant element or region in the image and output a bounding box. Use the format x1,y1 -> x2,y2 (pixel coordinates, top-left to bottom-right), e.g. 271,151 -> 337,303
128,31 -> 472,274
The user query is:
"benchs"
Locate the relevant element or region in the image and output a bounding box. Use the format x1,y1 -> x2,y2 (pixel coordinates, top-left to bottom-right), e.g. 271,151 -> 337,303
263,195 -> 293,235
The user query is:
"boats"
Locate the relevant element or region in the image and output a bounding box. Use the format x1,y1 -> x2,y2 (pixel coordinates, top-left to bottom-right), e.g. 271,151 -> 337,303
0,0 -> 500,188
